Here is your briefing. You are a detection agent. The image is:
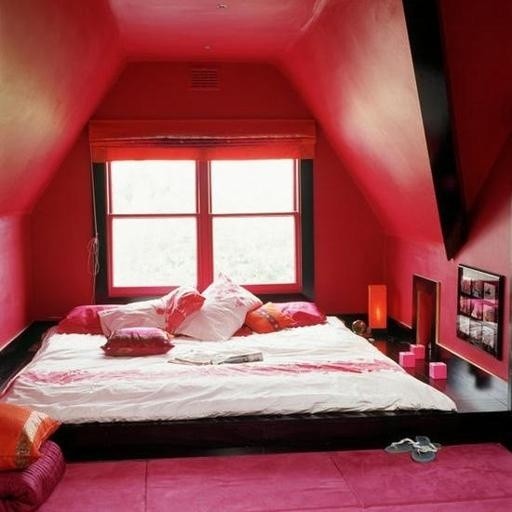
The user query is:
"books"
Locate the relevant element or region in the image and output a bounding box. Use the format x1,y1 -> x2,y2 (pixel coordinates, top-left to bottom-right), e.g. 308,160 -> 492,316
168,348 -> 263,364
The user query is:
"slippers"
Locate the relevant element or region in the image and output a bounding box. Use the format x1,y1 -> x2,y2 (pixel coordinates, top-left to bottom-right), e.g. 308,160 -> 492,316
411,435 -> 438,462
384,437 -> 442,453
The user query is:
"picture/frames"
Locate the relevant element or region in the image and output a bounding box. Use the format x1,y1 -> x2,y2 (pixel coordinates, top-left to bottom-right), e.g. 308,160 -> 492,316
454,263 -> 507,362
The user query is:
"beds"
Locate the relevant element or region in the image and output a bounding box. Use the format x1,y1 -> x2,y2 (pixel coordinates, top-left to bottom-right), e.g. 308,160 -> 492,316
1,311 -> 512,463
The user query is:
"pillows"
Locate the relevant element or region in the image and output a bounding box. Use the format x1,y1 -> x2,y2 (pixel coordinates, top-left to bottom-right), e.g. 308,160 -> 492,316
245,301 -> 290,333
97,285 -> 206,339
272,302 -> 328,329
4,441 -> 66,511
55,304 -> 128,334
0,402 -> 62,471
174,271 -> 263,342
100,327 -> 176,357
